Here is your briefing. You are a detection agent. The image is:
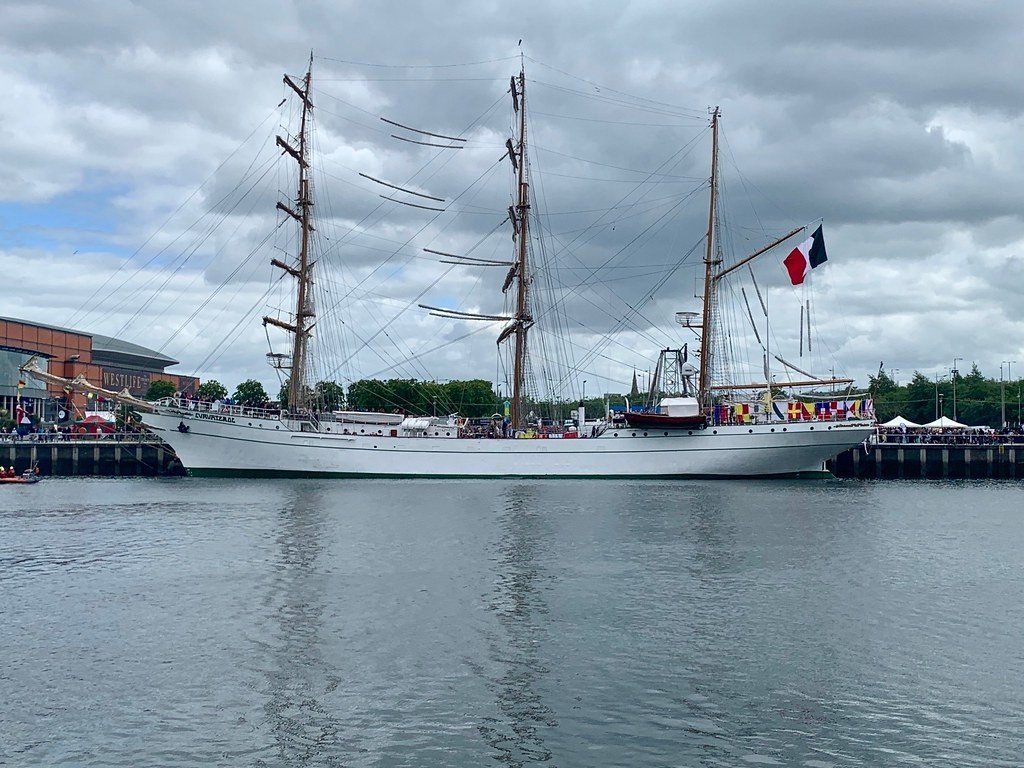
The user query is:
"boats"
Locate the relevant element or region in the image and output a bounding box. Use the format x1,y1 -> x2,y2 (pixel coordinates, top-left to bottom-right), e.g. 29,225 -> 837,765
0,468 -> 39,485
623,398 -> 708,430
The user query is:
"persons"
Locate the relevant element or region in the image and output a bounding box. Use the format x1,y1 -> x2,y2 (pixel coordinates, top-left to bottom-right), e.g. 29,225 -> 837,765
875,425 -> 1024,446
1,412 -> 144,481
173,384 -> 662,446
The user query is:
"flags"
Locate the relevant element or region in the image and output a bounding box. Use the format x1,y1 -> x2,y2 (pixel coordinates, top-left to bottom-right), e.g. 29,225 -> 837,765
783,224 -> 828,285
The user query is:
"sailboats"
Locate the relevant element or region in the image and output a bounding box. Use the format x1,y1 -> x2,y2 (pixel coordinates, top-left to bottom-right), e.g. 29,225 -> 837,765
13,36 -> 880,479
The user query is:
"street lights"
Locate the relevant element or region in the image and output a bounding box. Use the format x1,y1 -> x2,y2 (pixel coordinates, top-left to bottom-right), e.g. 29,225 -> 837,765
998,360 -> 1017,431
952,358 -> 963,424
